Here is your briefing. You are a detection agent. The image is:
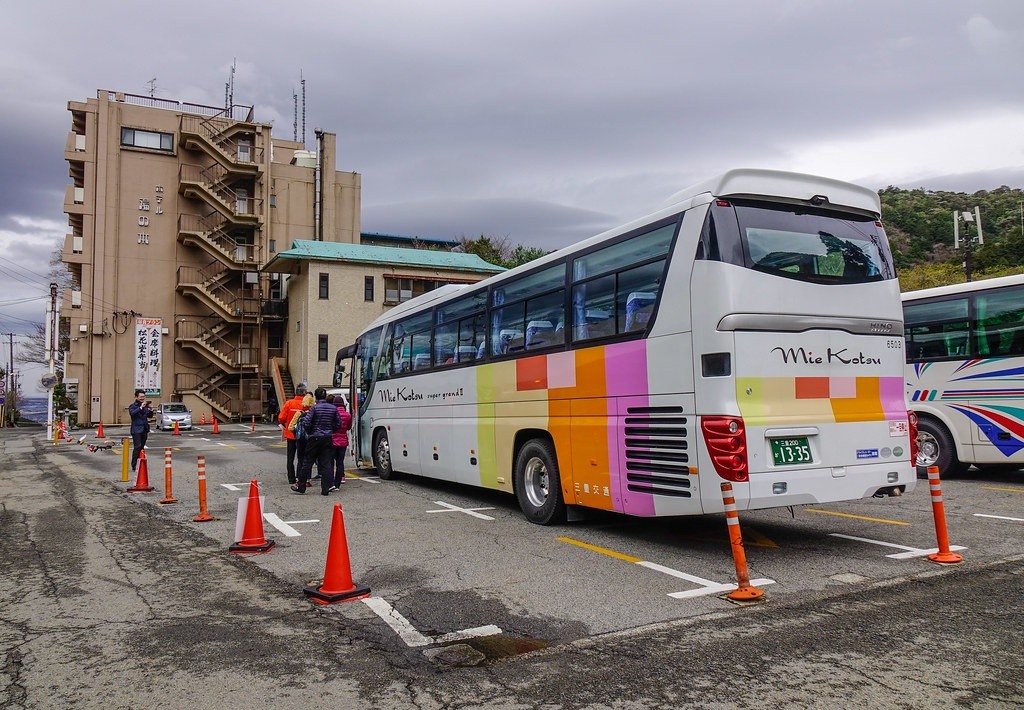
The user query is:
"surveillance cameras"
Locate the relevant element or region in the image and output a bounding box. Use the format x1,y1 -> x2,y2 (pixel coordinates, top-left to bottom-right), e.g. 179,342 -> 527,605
962,212 -> 974,224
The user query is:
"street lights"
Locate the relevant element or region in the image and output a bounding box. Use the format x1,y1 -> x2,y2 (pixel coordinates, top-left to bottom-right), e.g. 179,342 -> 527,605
954,206 -> 983,282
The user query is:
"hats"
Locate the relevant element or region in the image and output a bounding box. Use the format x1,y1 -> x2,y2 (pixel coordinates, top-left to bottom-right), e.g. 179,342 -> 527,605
333,396 -> 344,406
302,393 -> 315,407
326,395 -> 334,403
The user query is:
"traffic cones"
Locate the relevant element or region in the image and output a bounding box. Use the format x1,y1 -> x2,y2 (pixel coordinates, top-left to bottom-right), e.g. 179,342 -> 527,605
200,412 -> 206,425
127,449 -> 155,493
95,421 -> 105,438
211,419 -> 221,434
229,480 -> 275,554
303,504 -> 371,605
210,413 -> 214,424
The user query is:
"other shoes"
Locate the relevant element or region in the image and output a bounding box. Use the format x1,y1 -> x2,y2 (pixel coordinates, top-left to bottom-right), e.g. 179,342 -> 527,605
295,481 -> 299,486
291,486 -> 305,493
313,474 -> 322,479
341,477 -> 345,484
328,482 -> 336,491
333,488 -> 340,491
321,489 -> 329,496
131,464 -> 136,471
289,480 -> 297,484
306,481 -> 312,487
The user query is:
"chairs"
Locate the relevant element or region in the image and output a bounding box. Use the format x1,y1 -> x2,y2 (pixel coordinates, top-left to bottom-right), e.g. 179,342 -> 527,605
905,328 -> 1024,359
414,292 -> 656,370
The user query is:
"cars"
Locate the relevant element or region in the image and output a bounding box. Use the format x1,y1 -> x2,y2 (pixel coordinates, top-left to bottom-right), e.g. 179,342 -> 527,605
156,403 -> 192,431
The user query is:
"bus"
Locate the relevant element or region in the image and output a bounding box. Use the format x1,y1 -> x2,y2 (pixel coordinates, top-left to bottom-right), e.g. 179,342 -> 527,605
326,389 -> 366,413
900,274 -> 1024,480
333,168 -> 917,525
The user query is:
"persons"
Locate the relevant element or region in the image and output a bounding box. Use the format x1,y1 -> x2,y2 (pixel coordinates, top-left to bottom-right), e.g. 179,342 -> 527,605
442,353 -> 456,364
291,388 -> 341,495
278,383 -> 307,485
288,394 -> 315,482
327,395 -> 335,404
329,396 -> 352,491
128,389 -> 154,472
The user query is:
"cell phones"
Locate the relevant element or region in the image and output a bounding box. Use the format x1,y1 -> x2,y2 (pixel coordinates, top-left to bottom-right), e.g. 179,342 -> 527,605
146,401 -> 152,404
